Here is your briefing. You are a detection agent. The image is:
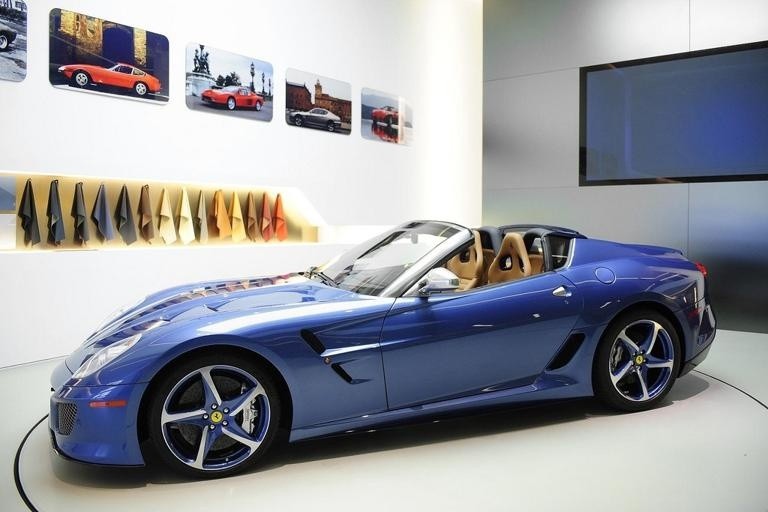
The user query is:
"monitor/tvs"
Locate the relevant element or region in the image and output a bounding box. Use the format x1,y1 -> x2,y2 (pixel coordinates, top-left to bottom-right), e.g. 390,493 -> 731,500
579,39 -> 768,190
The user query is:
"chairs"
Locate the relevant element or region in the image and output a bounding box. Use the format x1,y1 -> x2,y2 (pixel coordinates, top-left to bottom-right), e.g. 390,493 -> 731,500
444,230 -> 531,295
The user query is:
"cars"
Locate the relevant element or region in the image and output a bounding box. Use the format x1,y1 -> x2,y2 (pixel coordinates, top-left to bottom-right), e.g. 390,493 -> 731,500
371,106 -> 399,128
199,84 -> 265,112
288,106 -> 343,133
0,18 -> 19,56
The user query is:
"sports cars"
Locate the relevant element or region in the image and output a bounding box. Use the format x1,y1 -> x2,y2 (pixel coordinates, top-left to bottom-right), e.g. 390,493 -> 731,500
38,217 -> 715,483
54,59 -> 166,98
